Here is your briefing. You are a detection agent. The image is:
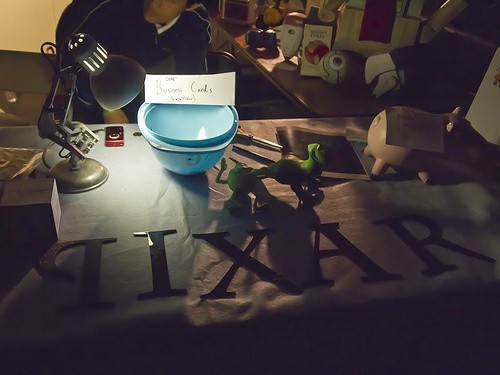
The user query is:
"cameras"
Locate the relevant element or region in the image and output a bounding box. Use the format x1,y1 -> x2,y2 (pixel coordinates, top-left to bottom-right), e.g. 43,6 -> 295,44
105,126 -> 124,147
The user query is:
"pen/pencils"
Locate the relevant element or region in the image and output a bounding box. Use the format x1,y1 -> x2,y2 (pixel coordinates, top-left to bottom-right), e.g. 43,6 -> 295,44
232,145 -> 277,165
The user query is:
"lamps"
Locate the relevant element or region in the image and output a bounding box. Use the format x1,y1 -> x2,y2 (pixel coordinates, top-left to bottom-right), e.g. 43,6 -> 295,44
38,33 -> 147,192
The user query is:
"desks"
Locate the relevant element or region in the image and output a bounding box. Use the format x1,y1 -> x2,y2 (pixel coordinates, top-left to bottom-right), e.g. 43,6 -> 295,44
0,116 -> 500,375
200,0 -> 422,116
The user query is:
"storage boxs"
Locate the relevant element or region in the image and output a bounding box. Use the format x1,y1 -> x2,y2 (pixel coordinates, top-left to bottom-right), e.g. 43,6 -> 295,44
0,178 -> 62,244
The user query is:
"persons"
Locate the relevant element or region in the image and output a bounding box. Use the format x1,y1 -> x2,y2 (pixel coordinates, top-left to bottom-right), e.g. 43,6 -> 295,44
58,0 -> 212,127
